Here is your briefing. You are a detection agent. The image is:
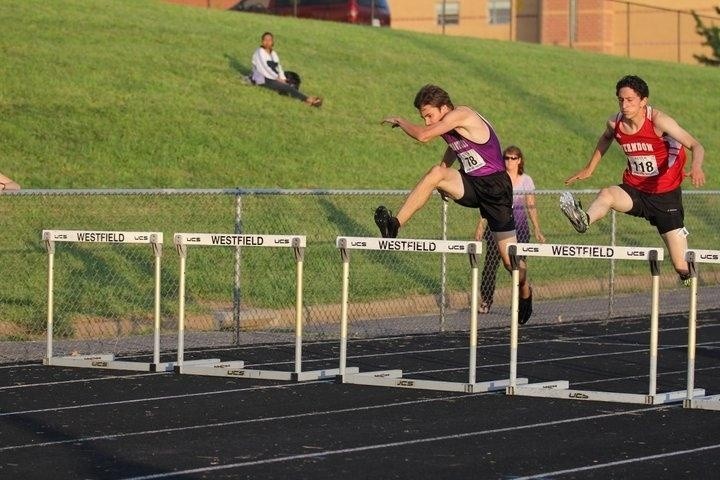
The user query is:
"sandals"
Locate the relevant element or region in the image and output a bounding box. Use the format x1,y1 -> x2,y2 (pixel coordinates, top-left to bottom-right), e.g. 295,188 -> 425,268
479,298 -> 493,313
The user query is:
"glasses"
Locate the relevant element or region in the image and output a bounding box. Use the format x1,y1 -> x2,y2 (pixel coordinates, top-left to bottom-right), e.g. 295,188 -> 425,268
504,156 -> 519,161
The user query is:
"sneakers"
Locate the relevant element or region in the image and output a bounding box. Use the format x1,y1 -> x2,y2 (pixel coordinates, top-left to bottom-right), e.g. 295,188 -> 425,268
518,288 -> 532,326
374,207 -> 399,238
681,262 -> 695,289
312,96 -> 323,106
559,193 -> 590,234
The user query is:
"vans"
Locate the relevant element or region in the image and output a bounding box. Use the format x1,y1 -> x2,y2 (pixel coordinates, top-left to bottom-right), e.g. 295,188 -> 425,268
227,0 -> 390,28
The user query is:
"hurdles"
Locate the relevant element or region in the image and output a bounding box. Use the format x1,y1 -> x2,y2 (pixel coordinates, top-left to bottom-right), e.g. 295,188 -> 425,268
173,233 -> 360,383
505,243 -> 706,404
42,230 -> 221,373
683,248 -> 719,411
336,236 -> 529,393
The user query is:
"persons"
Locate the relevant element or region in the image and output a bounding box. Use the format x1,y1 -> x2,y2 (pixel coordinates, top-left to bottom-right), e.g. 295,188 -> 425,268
372,83 -> 536,326
557,75 -> 709,298
250,31 -> 325,109
474,145 -> 546,315
